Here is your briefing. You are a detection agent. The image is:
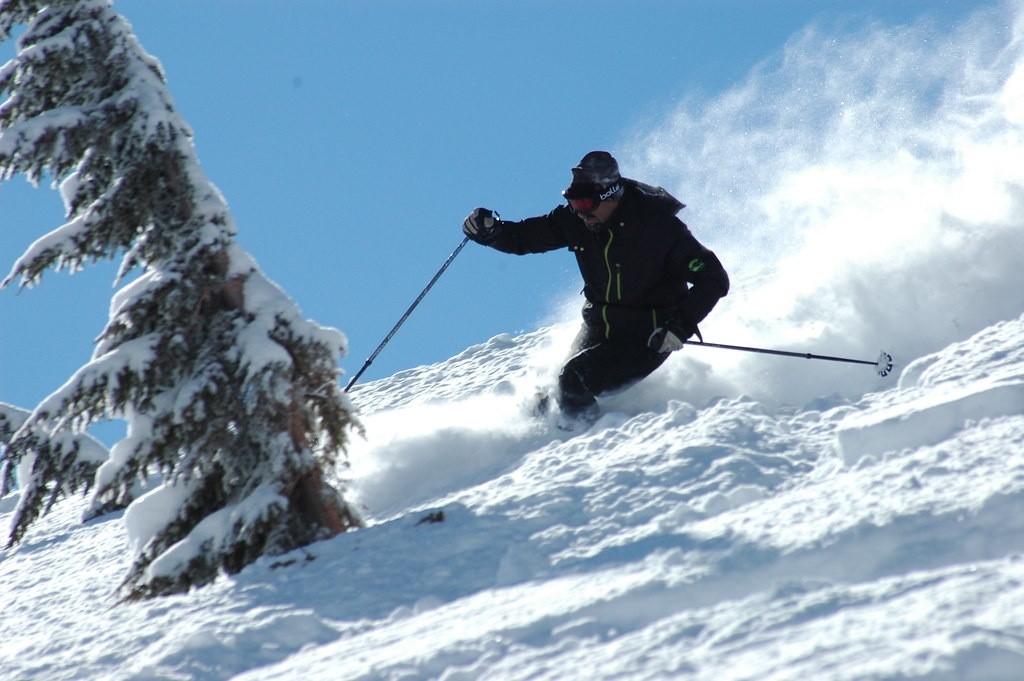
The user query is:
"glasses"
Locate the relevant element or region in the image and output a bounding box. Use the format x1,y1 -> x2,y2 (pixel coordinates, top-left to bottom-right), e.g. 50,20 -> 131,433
569,202 -> 603,221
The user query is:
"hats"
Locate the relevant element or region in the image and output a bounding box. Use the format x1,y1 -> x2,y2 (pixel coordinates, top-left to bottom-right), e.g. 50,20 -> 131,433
564,151 -> 625,201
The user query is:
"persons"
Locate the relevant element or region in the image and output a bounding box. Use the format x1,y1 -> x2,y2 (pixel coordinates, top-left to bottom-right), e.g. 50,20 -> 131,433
463,151 -> 729,441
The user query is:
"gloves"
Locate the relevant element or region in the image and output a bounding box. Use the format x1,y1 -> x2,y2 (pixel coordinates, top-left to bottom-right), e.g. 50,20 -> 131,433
647,312 -> 696,354
463,208 -> 513,247
